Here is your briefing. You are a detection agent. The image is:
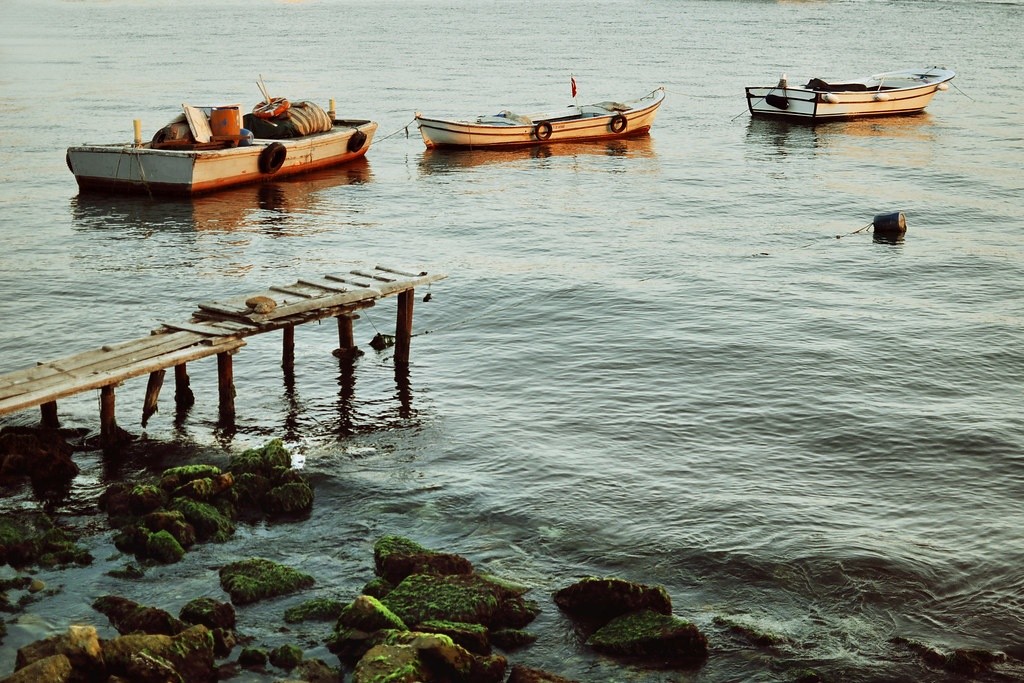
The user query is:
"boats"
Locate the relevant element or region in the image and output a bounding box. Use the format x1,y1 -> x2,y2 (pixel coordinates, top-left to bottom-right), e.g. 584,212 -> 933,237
745,67 -> 956,121
414,85 -> 665,150
65,119 -> 378,199
